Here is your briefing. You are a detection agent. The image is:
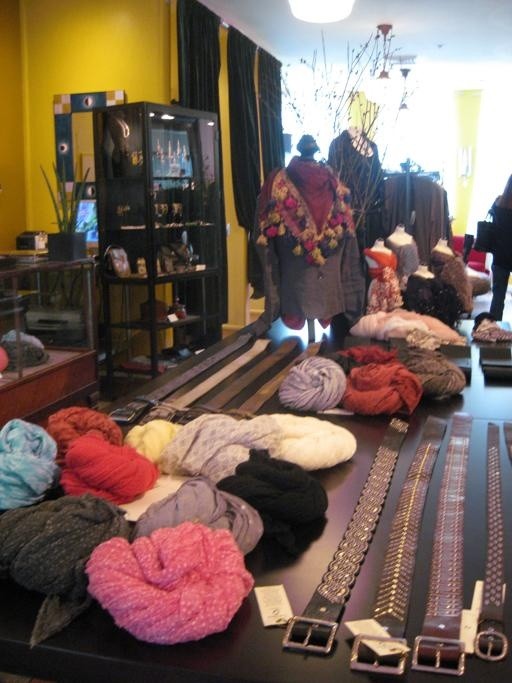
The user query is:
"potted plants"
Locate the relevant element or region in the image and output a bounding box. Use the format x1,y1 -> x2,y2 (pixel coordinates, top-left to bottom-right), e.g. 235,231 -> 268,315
39,154 -> 90,260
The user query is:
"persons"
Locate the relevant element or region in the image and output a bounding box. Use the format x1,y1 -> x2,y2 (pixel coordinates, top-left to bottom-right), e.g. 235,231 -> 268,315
490,167 -> 512,322
330,124 -> 384,249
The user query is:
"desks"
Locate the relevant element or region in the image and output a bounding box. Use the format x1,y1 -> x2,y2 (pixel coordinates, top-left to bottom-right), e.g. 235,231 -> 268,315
0,286 -> 512,683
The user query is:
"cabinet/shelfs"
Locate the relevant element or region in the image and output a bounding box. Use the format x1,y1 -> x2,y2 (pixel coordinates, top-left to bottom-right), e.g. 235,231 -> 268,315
0,249 -> 98,429
94,101 -> 223,400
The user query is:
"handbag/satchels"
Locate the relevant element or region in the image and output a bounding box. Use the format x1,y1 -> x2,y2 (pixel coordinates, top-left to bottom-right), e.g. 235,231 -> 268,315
473,196 -> 501,253
